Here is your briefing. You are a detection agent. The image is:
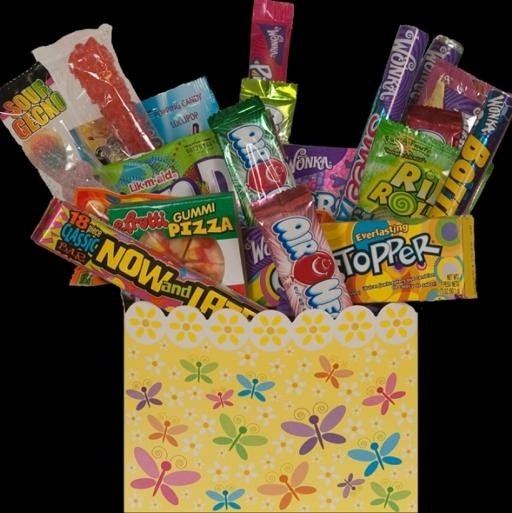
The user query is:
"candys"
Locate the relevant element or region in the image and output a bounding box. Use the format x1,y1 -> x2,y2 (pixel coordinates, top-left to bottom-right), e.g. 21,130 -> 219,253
239,158 -> 285,194
282,250 -> 335,286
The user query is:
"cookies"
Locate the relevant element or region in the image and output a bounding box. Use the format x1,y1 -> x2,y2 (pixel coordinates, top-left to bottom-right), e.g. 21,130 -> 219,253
170,236 -> 224,284
